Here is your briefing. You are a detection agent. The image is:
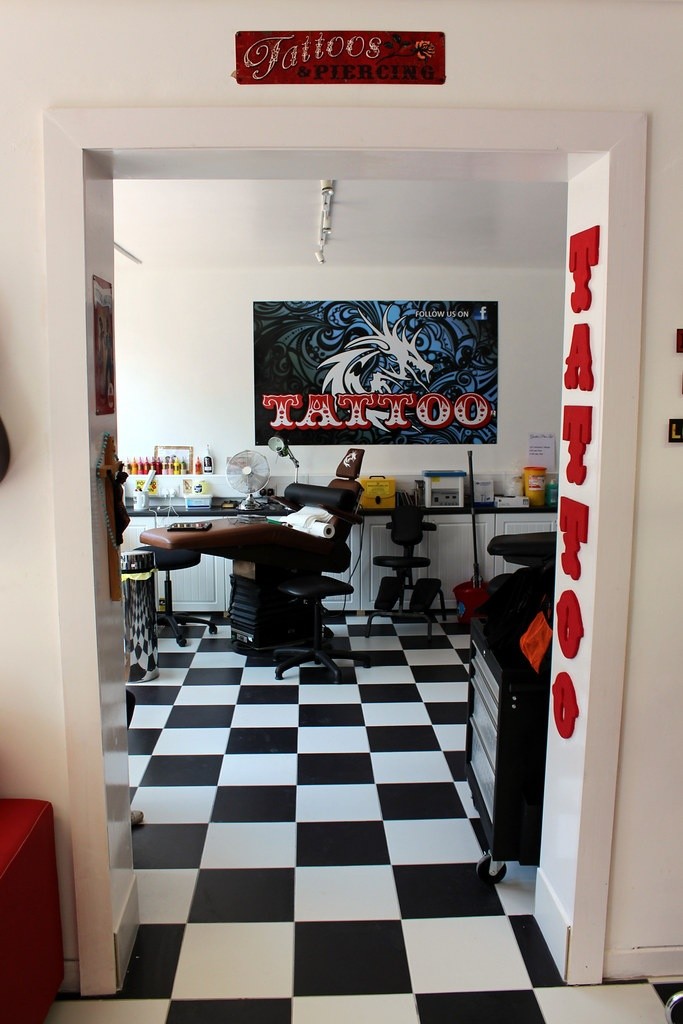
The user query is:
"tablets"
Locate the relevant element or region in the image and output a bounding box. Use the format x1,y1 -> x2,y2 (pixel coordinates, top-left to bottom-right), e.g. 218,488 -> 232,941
167,522 -> 212,532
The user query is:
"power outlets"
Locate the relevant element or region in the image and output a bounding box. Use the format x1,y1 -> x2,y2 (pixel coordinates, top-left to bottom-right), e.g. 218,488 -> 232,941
257,482 -> 277,497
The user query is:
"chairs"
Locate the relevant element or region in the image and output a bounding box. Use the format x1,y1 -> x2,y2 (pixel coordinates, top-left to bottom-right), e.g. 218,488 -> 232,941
364,505 -> 447,641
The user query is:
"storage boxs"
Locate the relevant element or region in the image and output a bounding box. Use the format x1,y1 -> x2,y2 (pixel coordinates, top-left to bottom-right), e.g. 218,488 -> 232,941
494,496 -> 530,508
184,495 -> 214,509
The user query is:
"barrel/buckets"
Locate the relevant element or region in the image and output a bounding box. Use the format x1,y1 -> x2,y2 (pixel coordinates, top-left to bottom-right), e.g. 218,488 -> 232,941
452,579 -> 490,624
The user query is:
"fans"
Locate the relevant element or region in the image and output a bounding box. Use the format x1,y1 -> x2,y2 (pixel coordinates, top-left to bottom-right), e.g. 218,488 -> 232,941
225,450 -> 271,510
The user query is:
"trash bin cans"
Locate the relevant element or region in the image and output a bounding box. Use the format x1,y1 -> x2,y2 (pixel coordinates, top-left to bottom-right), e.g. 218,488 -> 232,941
118,550 -> 161,684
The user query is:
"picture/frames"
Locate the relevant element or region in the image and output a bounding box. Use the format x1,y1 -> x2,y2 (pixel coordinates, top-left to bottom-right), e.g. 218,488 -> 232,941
153,444 -> 194,474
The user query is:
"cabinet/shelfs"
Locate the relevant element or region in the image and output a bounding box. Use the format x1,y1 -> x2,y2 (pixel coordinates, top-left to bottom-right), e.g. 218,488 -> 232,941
120,493 -> 556,613
462,610 -> 553,884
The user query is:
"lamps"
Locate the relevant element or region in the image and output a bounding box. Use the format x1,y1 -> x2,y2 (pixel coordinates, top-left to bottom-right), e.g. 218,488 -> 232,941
267,428 -> 300,482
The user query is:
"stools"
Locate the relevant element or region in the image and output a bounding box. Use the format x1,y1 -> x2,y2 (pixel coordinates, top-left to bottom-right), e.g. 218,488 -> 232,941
0,796 -> 66,1024
133,545 -> 218,647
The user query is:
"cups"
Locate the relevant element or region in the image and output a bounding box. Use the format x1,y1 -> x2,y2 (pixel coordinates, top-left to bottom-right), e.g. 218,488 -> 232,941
133,489 -> 149,510
523,466 -> 547,506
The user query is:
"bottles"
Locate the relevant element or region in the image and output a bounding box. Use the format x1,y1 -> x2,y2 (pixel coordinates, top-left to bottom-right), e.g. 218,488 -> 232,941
546,478 -> 558,505
